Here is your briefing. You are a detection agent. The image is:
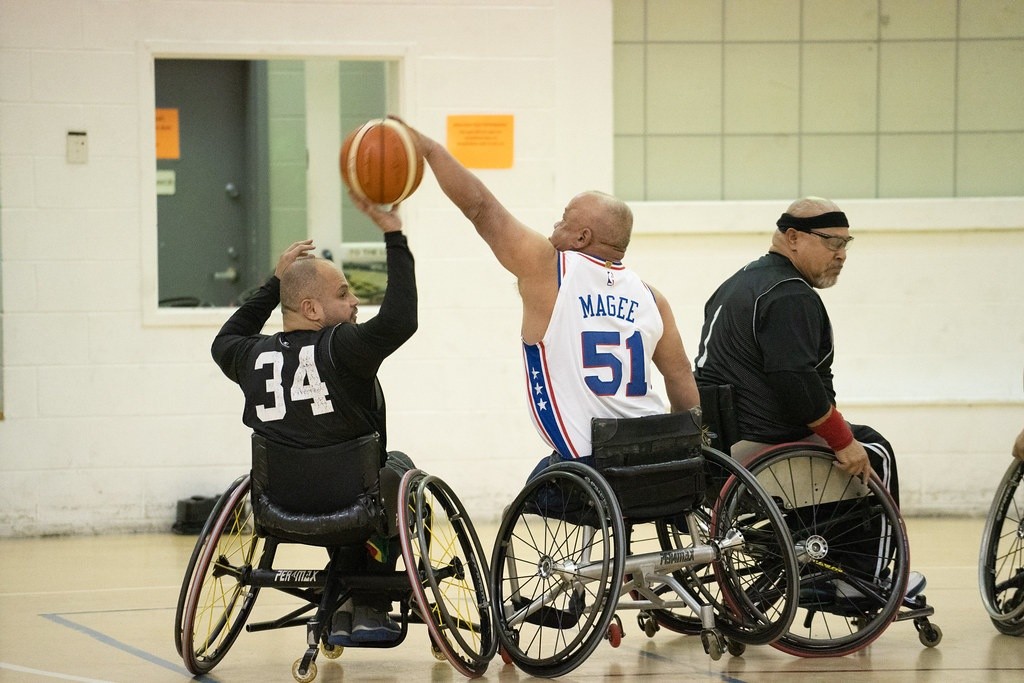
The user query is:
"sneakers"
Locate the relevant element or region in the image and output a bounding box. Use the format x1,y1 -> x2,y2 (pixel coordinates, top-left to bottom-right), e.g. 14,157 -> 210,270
351,605 -> 400,642
329,611 -> 358,647
798,573 -> 836,601
834,570 -> 926,613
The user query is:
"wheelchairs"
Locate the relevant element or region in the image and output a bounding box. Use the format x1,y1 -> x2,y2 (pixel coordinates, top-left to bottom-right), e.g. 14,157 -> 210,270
977,451 -> 1024,637
173,430 -> 499,683
489,400 -> 801,680
635,382 -> 944,649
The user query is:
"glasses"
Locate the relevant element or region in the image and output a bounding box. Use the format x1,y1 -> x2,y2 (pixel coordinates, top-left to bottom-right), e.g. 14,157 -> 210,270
804,230 -> 855,251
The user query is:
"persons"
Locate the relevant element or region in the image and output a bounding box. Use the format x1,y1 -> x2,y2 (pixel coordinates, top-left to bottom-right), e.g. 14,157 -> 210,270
384,116 -> 703,517
693,192 -> 928,615
207,204 -> 421,646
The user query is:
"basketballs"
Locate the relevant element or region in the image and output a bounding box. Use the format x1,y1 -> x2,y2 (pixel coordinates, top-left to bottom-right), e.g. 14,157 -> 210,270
340,118 -> 424,206
